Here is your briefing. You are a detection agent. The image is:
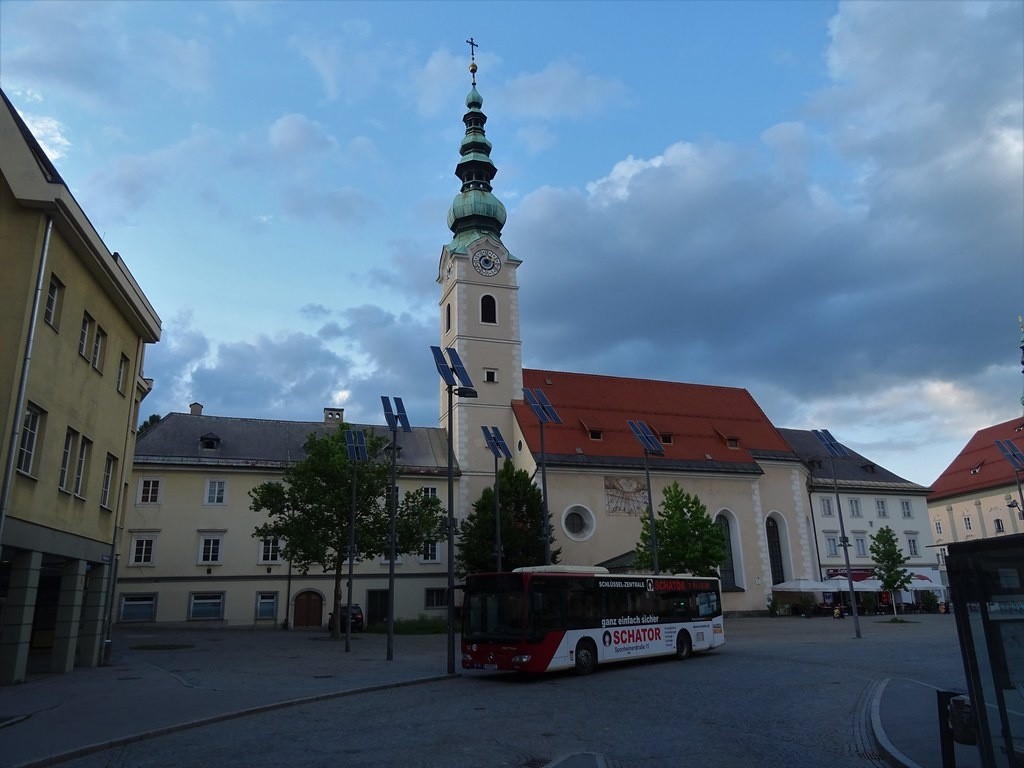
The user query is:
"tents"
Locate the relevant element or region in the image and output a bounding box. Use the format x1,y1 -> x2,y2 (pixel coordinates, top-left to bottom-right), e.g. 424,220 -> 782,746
770,576 -> 840,618
823,574 -> 947,616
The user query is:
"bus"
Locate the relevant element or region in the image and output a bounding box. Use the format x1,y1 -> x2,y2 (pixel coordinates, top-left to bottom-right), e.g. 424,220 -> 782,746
440,564 -> 727,677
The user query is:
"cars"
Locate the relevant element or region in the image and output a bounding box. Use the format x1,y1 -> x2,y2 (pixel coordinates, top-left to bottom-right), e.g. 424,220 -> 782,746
328,602 -> 364,633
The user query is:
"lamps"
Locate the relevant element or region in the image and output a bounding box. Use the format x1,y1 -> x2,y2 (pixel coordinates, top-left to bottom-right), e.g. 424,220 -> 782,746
1006,500 -> 1021,512
471,249 -> 501,277
